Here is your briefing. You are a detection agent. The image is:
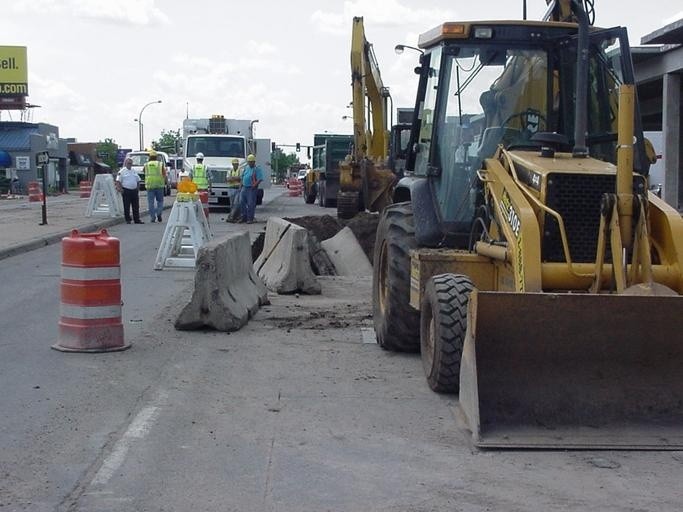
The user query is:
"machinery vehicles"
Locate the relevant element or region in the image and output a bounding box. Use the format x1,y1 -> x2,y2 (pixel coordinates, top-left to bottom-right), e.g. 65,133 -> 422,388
336,16 -> 415,220
302,133 -> 354,207
286,163 -> 307,188
372,1 -> 682,454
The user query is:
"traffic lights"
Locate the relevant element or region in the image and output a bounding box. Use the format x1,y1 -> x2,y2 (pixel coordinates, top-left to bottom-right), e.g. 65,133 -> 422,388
295,143 -> 299,152
272,142 -> 274,150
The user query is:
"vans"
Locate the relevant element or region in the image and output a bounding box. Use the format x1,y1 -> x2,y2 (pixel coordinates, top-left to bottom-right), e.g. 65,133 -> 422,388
297,170 -> 309,190
117,150 -> 172,196
168,157 -> 182,188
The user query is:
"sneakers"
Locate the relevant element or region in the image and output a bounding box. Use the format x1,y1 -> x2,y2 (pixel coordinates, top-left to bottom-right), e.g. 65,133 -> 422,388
126,221 -> 131,224
158,215 -> 161,221
135,221 -> 144,224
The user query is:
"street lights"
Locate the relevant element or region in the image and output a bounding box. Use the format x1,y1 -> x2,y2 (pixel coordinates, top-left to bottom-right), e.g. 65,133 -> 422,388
341,101 -> 374,135
139,100 -> 161,151
134,118 -> 143,150
395,43 -> 425,57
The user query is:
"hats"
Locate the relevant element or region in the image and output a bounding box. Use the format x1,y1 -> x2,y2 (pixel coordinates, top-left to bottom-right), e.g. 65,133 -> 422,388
232,159 -> 239,164
150,151 -> 157,156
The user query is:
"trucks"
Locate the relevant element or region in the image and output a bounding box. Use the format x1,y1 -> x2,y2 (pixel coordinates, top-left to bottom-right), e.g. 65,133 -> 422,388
176,133 -> 270,213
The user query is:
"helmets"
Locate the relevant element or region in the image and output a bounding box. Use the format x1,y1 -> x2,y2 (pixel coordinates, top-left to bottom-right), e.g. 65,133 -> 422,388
247,154 -> 256,162
196,153 -> 204,160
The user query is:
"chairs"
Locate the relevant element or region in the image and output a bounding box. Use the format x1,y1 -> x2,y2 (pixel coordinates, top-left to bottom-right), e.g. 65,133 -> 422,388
476,126 -> 521,156
229,143 -> 241,157
196,143 -> 204,152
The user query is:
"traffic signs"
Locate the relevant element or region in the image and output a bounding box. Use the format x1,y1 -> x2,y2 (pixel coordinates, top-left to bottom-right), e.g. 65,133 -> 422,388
35,150 -> 49,166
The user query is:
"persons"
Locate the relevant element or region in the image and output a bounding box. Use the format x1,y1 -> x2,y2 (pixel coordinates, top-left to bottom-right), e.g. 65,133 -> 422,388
237,154 -> 265,223
116,157 -> 143,224
226,159 -> 244,223
143,150 -> 168,222
189,152 -> 214,191
